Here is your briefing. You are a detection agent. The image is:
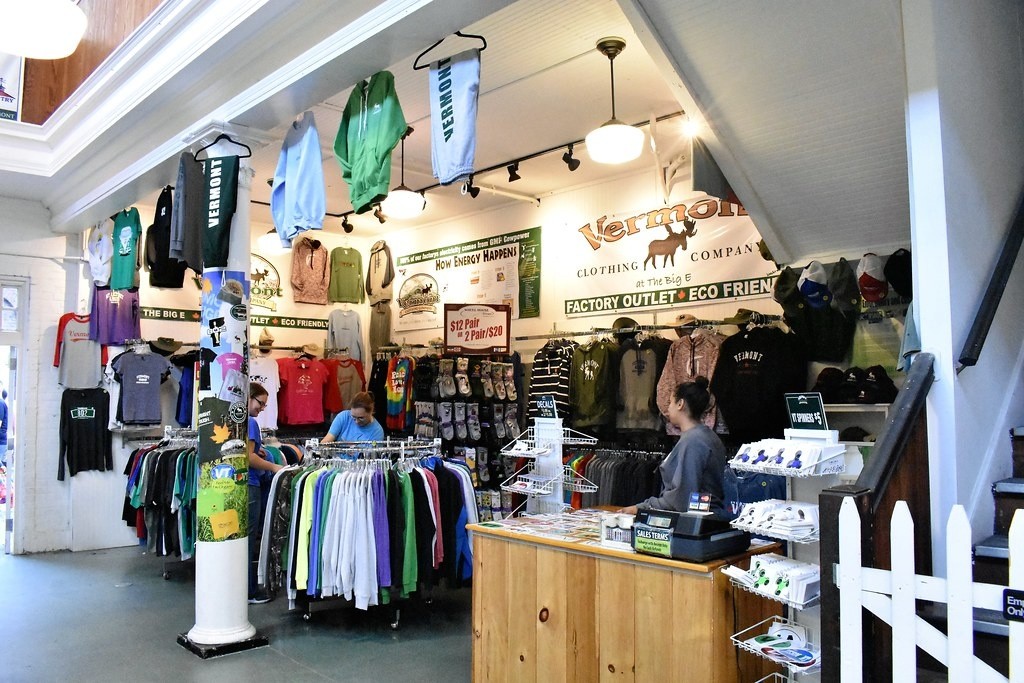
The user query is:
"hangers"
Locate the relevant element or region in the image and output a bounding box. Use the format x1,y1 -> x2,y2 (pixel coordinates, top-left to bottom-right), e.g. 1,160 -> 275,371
413,31 -> 487,69
563,436 -> 672,463
194,121 -> 252,162
124,340 -> 515,628
547,309 -> 778,352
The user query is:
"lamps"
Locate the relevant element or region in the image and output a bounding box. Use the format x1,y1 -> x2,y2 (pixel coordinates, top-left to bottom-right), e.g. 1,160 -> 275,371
341,214 -> 354,234
460,175 -> 480,198
0,0 -> 88,60
562,144 -> 580,171
507,162 -> 521,182
374,204 -> 389,224
418,190 -> 427,210
258,177 -> 295,254
585,36 -> 644,165
380,125 -> 425,218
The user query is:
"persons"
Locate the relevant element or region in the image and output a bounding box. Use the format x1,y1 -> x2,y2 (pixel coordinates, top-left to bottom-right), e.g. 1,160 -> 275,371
617,375 -> 726,515
320,390 -> 385,460
248,382 -> 283,603
0,380 -> 8,504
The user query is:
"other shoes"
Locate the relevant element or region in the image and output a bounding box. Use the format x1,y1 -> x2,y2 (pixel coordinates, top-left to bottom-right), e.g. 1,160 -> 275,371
247,589 -> 271,605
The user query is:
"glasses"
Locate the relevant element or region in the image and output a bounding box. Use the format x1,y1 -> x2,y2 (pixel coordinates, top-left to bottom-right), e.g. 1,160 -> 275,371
351,415 -> 366,421
253,397 -> 267,408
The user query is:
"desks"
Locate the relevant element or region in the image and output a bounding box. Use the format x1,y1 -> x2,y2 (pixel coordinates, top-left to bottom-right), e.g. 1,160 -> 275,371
465,506 -> 783,683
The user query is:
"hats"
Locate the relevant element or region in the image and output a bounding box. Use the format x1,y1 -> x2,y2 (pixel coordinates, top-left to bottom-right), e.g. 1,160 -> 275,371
259,327 -> 274,353
773,266 -> 805,318
884,248 -> 912,297
840,426 -> 876,442
828,257 -> 860,319
149,337 -> 182,356
304,343 -> 324,356
612,317 -> 641,338
797,260 -> 832,309
810,365 -> 894,405
665,314 -> 699,338
856,253 -> 888,302
724,309 -> 761,330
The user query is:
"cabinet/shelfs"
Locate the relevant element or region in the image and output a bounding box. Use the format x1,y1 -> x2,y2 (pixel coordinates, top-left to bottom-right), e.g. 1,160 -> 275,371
823,403 -> 894,455
500,417 -> 599,520
722,437 -> 848,683
